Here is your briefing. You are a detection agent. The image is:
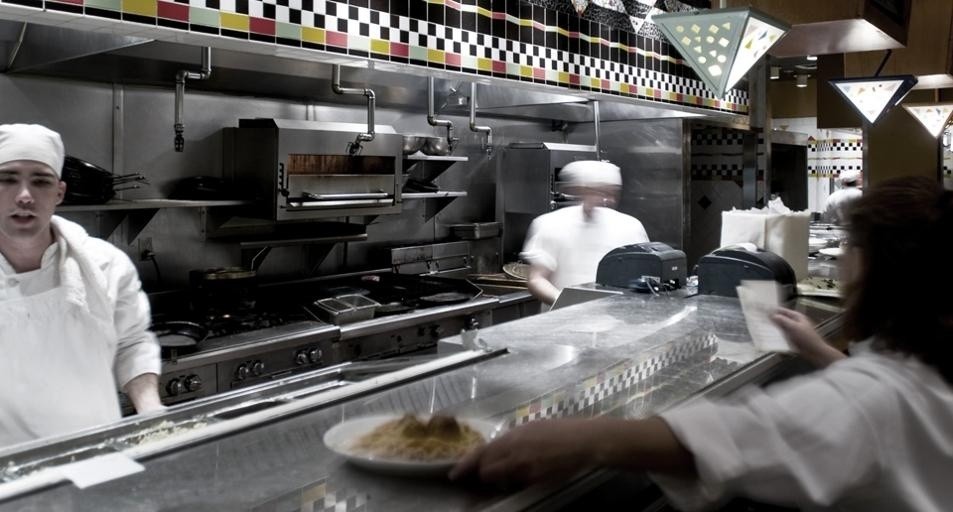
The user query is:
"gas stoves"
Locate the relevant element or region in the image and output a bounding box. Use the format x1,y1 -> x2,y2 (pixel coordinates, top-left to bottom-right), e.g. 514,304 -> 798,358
154,296 -> 341,407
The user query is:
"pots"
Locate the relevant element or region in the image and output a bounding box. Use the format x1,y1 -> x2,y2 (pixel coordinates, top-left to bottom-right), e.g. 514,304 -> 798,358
148,320 -> 209,367
61,156 -> 148,204
189,266 -> 257,310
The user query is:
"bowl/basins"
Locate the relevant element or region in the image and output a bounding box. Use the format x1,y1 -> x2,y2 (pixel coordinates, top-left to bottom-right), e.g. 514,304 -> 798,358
403,137 -> 460,155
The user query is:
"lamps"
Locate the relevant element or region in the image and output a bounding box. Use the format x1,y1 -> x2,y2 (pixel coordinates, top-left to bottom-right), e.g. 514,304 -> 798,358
651,0 -> 918,130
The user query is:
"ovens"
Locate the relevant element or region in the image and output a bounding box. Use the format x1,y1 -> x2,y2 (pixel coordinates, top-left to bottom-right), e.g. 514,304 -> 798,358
221,118 -> 402,222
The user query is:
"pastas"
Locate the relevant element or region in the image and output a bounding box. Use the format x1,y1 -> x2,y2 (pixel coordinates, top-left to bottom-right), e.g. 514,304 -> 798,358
357,419 -> 486,462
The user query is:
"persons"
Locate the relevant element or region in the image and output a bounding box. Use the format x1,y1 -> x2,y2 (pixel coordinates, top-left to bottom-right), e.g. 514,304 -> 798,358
482,174 -> 953,510
1,121 -> 167,455
518,160 -> 652,314
821,170 -> 865,230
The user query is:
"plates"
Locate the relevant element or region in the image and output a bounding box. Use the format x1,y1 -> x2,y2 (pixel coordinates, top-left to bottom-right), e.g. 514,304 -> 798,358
323,412 -> 500,477
809,235 -> 826,254
818,247 -> 845,258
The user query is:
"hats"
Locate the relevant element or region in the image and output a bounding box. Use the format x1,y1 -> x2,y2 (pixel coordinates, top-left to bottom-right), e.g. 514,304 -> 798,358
839,169 -> 859,183
560,160 -> 623,187
0,123 -> 66,180
